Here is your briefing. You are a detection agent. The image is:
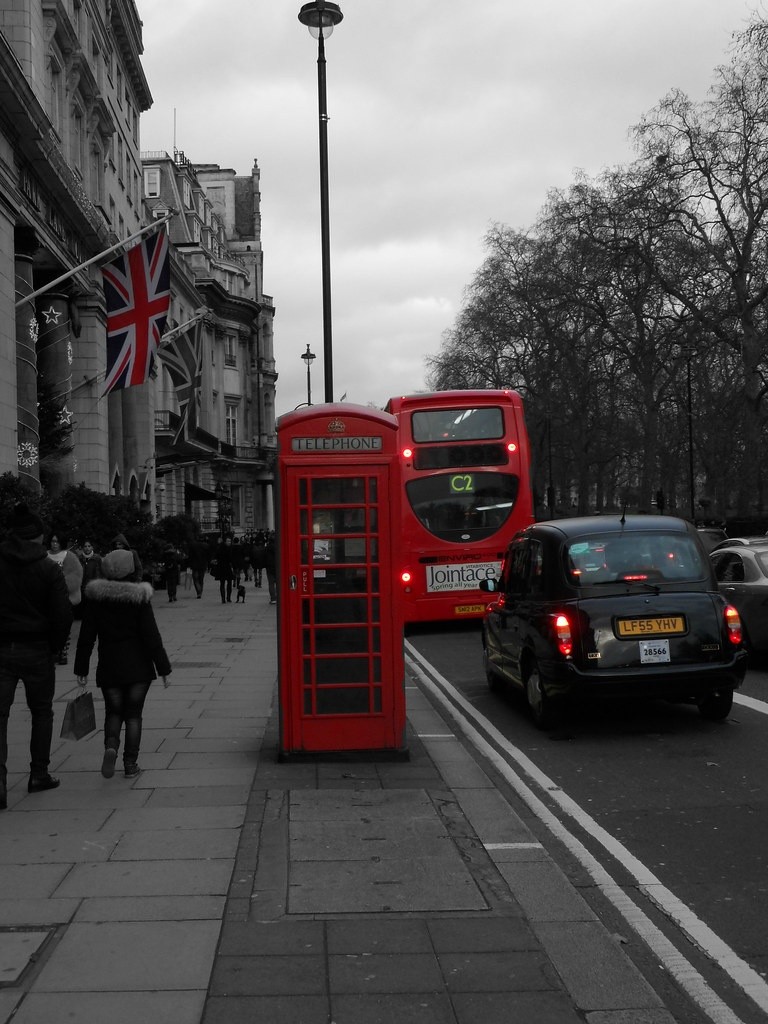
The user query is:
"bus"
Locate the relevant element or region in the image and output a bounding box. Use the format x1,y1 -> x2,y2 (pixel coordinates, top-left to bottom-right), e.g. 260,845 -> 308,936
385,389 -> 536,633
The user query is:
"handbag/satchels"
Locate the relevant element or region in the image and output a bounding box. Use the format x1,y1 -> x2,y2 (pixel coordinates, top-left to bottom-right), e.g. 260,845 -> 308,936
210,563 -> 218,577
60,680 -> 96,741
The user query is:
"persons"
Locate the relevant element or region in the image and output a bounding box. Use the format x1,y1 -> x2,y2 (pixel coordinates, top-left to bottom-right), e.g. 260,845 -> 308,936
1,527 -> 282,815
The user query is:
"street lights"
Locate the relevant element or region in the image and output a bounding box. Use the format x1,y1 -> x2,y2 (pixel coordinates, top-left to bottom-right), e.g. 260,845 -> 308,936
301,344 -> 317,405
544,405 -> 556,519
682,343 -> 698,522
298,0 -> 344,404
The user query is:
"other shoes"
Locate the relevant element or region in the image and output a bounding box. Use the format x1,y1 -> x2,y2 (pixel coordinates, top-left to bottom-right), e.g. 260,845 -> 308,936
27,773 -> 59,793
169,598 -> 173,602
255,580 -> 262,588
197,595 -> 201,599
59,657 -> 67,665
124,763 -> 141,778
174,597 -> 178,602
270,600 -> 276,604
101,748 -> 117,778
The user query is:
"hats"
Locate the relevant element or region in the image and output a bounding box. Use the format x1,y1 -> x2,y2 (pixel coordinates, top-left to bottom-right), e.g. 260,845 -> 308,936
14,503 -> 44,541
101,549 -> 135,579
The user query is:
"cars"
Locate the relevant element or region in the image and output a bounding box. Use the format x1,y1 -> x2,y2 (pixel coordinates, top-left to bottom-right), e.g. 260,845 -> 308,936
700,536 -> 768,670
480,516 -> 748,737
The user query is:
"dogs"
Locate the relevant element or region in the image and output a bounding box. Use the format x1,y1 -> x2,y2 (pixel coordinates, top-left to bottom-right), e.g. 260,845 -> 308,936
236,585 -> 246,603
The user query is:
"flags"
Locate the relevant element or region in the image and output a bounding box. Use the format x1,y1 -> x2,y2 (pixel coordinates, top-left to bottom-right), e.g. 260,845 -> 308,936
100,224 -> 172,401
